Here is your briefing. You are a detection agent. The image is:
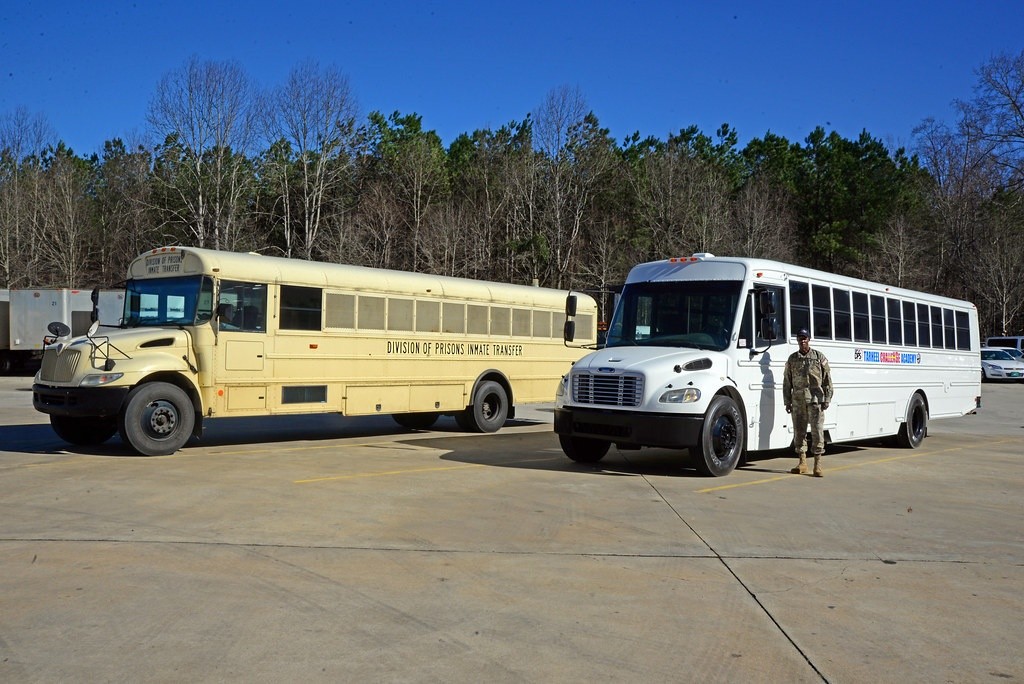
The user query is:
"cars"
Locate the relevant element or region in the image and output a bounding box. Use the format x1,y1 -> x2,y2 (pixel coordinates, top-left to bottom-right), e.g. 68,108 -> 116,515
981,348 -> 1024,383
988,346 -> 1024,363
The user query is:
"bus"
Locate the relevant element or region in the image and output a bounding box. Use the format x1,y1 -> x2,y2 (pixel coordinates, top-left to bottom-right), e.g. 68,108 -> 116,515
31,244 -> 599,457
551,253 -> 983,477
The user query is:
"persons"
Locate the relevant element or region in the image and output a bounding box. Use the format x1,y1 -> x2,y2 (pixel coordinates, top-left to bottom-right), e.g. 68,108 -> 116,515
783,330 -> 833,477
218,303 -> 241,329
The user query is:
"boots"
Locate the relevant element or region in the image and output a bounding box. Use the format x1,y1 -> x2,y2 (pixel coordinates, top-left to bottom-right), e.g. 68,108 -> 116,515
813,454 -> 823,476
791,453 -> 807,473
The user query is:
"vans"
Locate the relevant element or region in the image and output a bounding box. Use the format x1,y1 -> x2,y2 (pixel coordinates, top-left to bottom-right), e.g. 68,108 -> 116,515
986,336 -> 1024,353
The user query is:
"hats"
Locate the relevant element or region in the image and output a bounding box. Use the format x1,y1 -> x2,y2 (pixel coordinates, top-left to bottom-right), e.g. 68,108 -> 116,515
797,328 -> 809,337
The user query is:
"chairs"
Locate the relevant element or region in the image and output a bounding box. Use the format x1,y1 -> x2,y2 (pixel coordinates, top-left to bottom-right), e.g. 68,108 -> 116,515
217,303 -> 234,323
232,306 -> 258,329
